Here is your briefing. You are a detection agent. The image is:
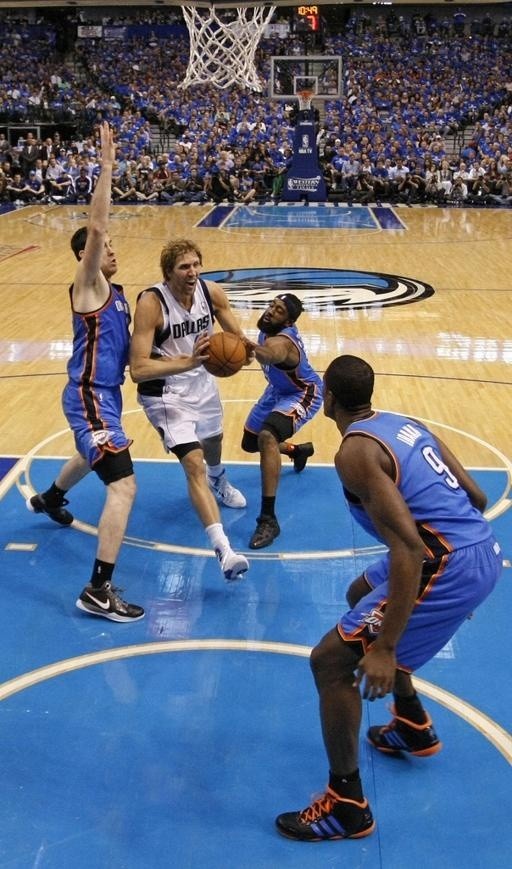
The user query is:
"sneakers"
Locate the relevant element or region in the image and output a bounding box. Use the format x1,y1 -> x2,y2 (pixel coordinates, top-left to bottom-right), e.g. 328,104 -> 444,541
30,494 -> 73,526
276,784 -> 376,841
208,469 -> 246,508
367,704 -> 442,757
293,443 -> 314,471
251,514 -> 280,549
213,543 -> 249,580
76,580 -> 145,623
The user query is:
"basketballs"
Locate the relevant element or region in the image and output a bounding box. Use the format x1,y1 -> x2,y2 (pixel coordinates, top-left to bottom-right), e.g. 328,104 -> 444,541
201,332 -> 245,377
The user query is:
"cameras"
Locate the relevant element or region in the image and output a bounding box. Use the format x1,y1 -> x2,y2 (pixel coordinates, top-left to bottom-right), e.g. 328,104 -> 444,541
431,175 -> 438,183
502,177 -> 506,181
406,177 -> 410,181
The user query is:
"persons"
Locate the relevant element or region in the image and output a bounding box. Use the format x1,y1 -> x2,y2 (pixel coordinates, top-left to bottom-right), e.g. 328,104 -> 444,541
130,238 -> 255,581
26,120 -> 145,623
242,294 -> 325,548
1,4 -> 512,206
275,355 -> 503,843
